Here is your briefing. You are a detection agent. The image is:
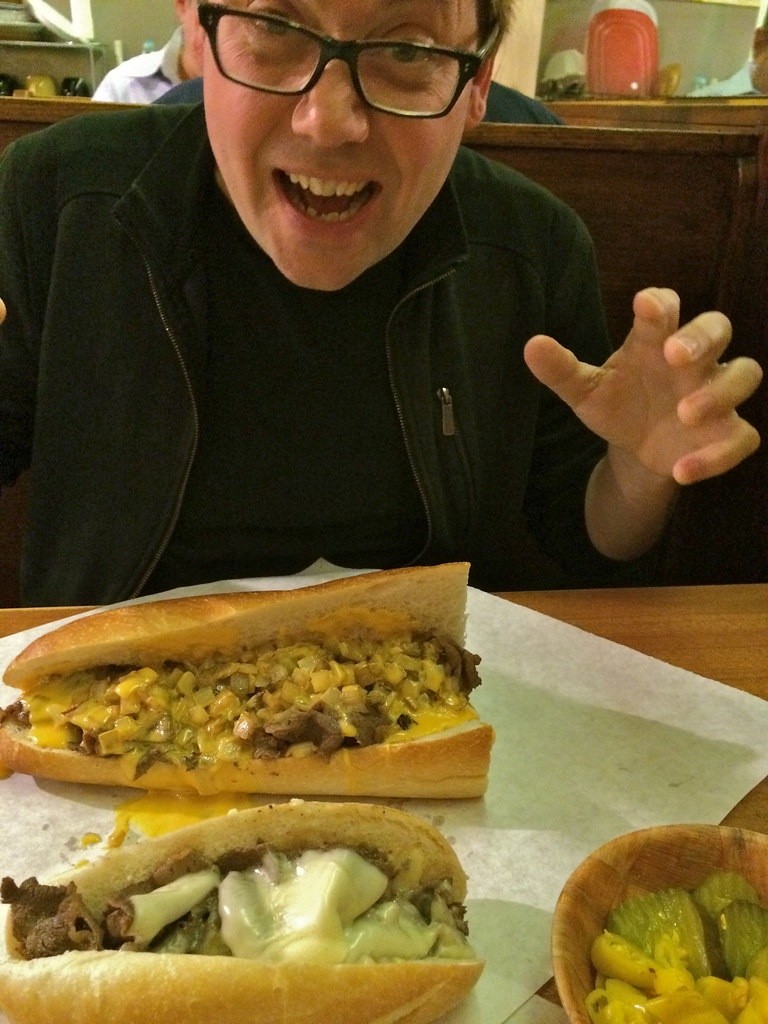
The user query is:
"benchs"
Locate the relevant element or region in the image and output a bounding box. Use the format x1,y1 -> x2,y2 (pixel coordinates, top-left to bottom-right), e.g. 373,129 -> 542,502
1,95 -> 767,587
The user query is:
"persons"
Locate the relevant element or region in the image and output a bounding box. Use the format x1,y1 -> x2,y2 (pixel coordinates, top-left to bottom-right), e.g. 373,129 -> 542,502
687,2 -> 768,99
89,0 -> 206,106
0,0 -> 764,606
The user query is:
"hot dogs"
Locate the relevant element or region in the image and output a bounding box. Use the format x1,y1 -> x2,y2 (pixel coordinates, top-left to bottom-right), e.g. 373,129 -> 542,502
1,563 -> 495,802
0,800 -> 488,1024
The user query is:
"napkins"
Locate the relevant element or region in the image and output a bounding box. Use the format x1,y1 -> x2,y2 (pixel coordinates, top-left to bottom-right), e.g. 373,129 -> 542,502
0,560 -> 767,1024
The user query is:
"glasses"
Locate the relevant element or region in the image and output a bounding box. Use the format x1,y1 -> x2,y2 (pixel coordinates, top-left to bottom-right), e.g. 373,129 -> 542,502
197,0 -> 501,119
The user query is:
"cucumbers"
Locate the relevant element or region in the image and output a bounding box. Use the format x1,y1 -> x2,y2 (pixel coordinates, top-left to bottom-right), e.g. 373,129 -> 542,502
605,868 -> 768,984
